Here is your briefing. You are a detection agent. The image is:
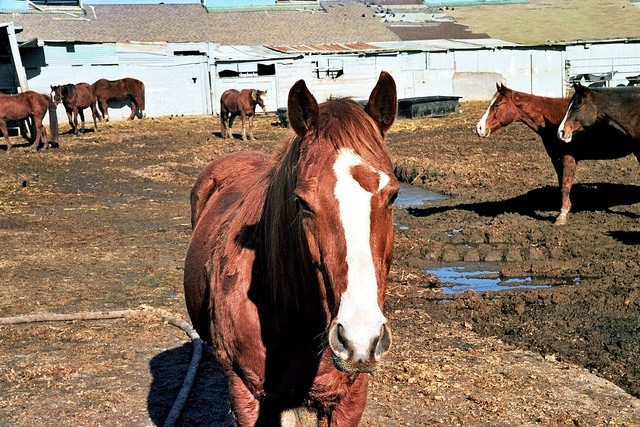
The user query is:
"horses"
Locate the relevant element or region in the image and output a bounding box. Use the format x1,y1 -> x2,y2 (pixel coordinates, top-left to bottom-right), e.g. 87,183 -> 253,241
0,90 -> 58,152
475,81 -> 640,226
220,88 -> 268,141
184,71 -> 400,427
91,77 -> 145,121
558,80 -> 640,150
50,82 -> 102,135
